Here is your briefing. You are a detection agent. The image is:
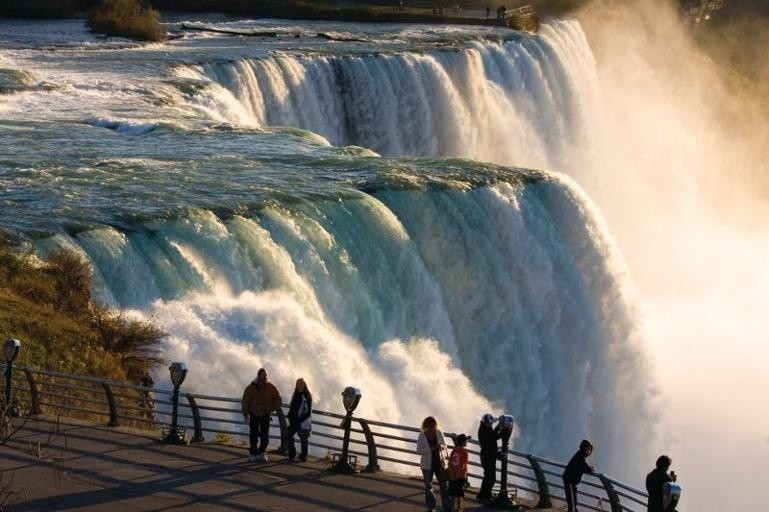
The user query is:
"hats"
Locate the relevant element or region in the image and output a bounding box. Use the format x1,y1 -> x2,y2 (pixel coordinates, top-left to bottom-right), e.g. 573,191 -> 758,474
455,433 -> 472,446
482,413 -> 500,425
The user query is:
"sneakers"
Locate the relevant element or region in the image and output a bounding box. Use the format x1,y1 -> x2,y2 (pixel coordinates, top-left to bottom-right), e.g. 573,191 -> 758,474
248,452 -> 308,464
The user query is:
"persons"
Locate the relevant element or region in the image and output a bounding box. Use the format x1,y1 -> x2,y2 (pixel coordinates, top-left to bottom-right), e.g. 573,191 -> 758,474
240,367 -> 283,462
645,454 -> 677,511
415,416 -> 452,511
283,377 -> 312,462
475,413 -> 503,504
562,439 -> 594,511
447,433 -> 470,511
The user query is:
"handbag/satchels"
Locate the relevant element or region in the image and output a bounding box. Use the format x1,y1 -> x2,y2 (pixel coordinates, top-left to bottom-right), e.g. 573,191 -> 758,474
437,468 -> 448,481
